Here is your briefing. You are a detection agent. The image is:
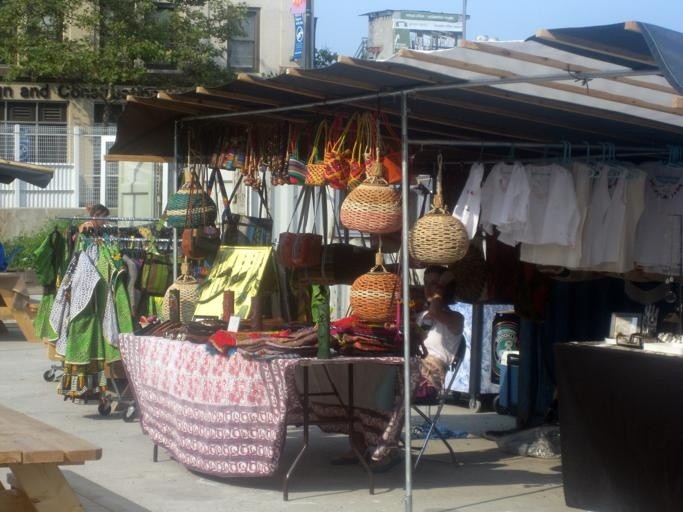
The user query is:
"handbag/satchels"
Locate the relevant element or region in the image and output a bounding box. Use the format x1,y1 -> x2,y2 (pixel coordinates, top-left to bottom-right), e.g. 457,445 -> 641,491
339,176 -> 403,234
208,112 -> 417,192
274,233 -> 375,289
219,208 -> 272,247
139,253 -> 181,297
409,209 -> 470,266
161,273 -> 202,325
159,174 -> 217,228
180,224 -> 220,260
349,266 -> 402,322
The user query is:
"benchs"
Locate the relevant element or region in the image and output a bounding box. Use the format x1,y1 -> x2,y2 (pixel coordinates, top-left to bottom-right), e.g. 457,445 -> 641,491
0,298 -> 40,343
0,400 -> 103,512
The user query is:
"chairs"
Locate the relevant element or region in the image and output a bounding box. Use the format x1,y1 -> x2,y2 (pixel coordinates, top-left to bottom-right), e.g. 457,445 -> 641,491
393,332 -> 463,477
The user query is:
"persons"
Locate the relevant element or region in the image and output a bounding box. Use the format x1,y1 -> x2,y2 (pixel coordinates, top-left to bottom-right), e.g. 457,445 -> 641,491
75,204 -> 110,234
327,264 -> 464,474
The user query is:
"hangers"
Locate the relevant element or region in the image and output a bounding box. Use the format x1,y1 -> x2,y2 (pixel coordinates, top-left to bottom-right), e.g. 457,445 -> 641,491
49,215 -> 62,241
67,214 -> 175,253
431,139 -> 683,187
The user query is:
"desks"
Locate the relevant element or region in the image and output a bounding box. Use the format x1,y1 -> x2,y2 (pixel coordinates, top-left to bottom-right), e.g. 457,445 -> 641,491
0,270 -> 36,305
554,334 -> 683,512
116,332 -> 417,501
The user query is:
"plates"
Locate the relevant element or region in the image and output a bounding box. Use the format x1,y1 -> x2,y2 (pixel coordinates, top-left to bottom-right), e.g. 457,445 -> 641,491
604,337 -> 615,344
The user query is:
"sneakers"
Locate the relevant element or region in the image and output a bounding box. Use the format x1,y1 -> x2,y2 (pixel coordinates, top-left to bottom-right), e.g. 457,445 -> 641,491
330,444 -> 402,469
